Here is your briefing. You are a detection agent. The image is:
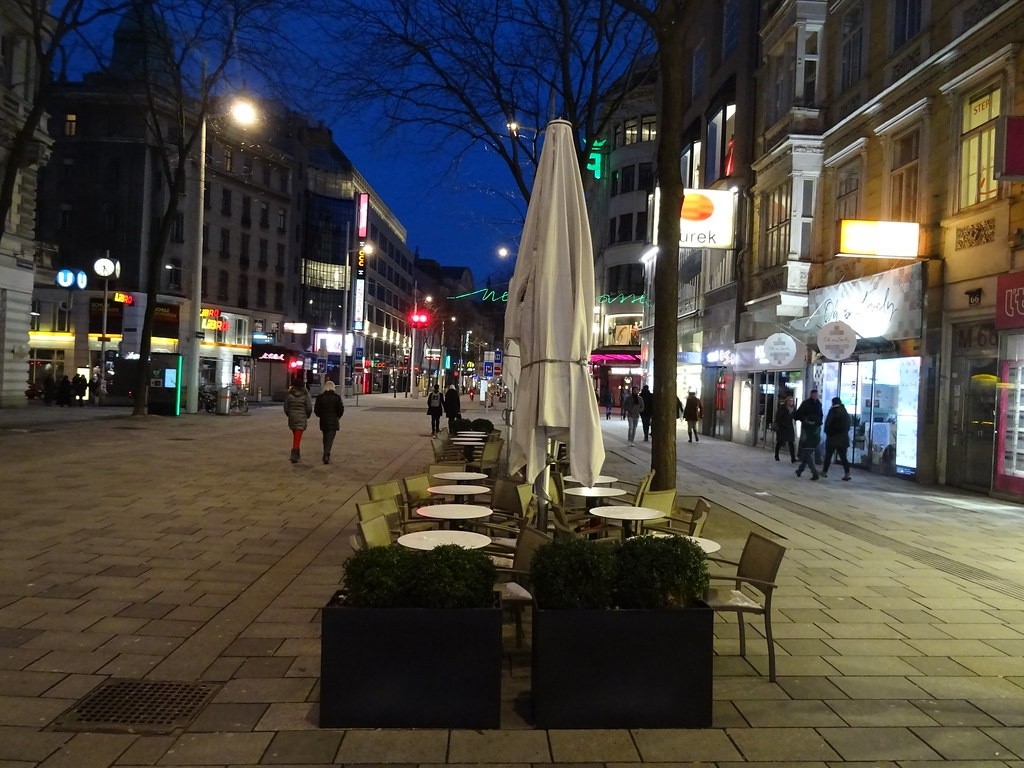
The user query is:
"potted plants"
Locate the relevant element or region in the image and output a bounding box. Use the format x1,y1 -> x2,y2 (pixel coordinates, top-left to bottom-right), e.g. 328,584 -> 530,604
529,534 -> 715,730
319,544 -> 501,731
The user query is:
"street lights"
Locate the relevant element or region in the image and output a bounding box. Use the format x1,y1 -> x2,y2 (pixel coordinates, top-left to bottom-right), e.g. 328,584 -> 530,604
439,317 -> 456,393
185,102 -> 255,414
339,247 -> 373,394
410,296 -> 432,393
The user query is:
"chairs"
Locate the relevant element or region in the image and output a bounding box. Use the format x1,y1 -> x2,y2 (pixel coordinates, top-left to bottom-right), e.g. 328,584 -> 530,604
707,532 -> 788,684
355,427 -> 710,646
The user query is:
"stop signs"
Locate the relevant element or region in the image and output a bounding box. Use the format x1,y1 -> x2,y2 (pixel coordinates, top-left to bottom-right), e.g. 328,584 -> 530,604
494,366 -> 501,375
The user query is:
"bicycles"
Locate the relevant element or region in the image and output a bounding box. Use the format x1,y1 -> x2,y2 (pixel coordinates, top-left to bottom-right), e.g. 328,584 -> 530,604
199,384 -> 249,416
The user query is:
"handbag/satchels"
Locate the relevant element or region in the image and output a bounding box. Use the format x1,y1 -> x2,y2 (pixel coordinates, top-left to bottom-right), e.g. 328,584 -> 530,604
771,422 -> 780,431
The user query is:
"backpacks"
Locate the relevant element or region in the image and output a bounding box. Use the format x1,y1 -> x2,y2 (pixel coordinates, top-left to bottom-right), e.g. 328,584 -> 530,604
431,392 -> 440,406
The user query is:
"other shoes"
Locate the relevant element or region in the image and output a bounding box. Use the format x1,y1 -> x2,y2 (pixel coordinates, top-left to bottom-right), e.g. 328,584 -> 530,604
643,438 -> 648,441
627,440 -> 634,447
841,474 -> 851,480
820,470 -> 827,477
796,470 -> 802,477
322,453 -> 330,464
810,475 -> 819,480
436,429 -> 442,432
431,432 -> 436,435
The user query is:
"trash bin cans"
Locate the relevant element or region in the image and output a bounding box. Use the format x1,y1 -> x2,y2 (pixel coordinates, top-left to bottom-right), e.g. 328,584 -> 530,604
217,388 -> 231,416
257,388 -> 263,402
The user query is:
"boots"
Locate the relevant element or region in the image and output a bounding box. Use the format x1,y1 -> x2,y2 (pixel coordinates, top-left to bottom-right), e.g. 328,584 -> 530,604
695,430 -> 699,441
791,456 -> 799,463
688,432 -> 692,442
775,453 -> 780,461
290,448 -> 300,463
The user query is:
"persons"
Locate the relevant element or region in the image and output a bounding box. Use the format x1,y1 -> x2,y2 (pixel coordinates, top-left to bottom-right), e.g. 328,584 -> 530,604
640,385 -> 653,442
503,115 -> 606,533
817,397 -> 852,481
314,381 -> 344,463
760,393 -> 774,440
694,162 -> 700,188
283,377 -> 313,463
444,384 -> 460,434
607,322 -> 616,344
631,320 -> 642,346
792,389 -> 824,481
624,386 -> 645,447
44,372 -> 86,408
725,134 -> 735,176
775,396 -> 798,463
676,396 -> 683,419
426,384 -> 444,439
683,392 -> 703,442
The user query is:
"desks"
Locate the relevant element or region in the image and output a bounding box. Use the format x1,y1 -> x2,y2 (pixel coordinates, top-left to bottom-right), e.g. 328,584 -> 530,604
562,488 -> 627,515
397,530 -> 490,552
427,484 -> 491,504
433,472 -> 488,485
457,433 -> 488,437
452,441 -> 485,463
563,475 -> 617,486
588,505 -> 665,537
450,437 -> 483,442
457,431 -> 486,435
627,534 -> 721,554
415,504 -> 493,530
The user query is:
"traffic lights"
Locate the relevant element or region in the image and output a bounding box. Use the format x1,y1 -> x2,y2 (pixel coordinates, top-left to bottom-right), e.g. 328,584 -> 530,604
410,314 -> 429,323
291,356 -> 297,370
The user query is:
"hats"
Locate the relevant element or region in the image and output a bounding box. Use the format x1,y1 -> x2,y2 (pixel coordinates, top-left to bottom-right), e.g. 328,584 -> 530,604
449,385 -> 455,389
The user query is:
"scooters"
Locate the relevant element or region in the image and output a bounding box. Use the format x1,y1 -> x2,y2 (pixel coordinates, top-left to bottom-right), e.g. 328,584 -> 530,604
470,393 -> 474,400
